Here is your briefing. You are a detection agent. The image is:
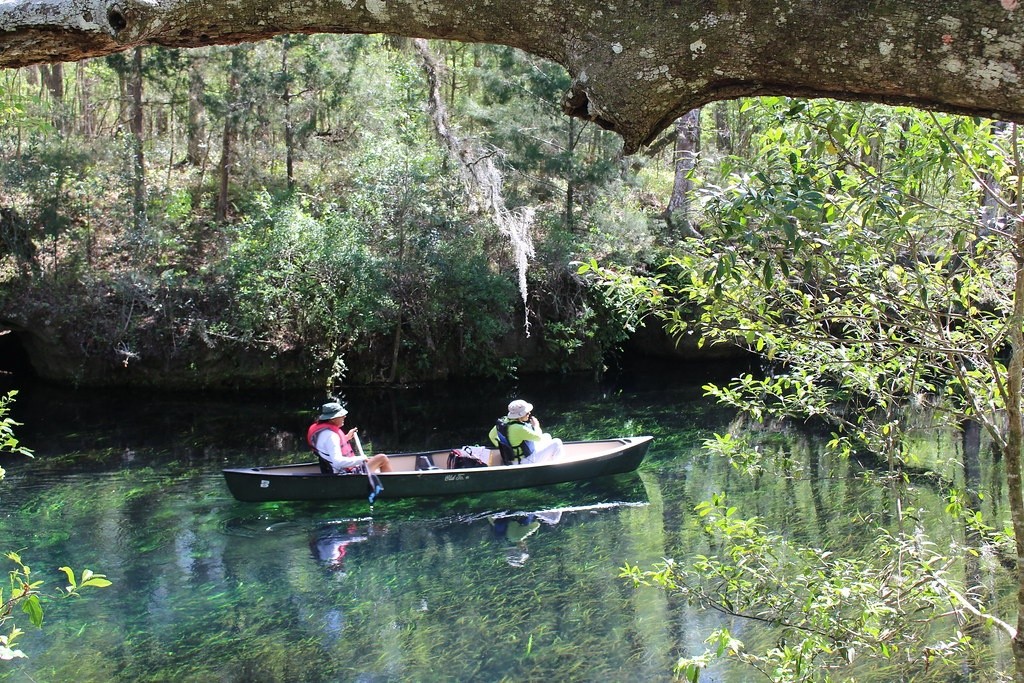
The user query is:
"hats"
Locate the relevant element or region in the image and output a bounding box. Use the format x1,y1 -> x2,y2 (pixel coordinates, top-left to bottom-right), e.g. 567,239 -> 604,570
507,400 -> 534,419
319,402 -> 349,420
507,549 -> 530,567
324,572 -> 347,583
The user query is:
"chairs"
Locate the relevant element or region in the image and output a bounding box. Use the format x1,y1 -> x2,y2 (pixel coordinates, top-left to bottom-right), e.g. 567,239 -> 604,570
318,455 -> 335,474
495,437 -> 515,465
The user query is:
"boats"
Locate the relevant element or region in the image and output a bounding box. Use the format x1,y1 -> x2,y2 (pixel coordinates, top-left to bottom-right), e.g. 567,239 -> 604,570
226,469 -> 650,539
222,436 -> 655,505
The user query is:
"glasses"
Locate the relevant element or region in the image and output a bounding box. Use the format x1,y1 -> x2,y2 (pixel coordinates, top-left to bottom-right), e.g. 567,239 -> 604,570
526,412 -> 531,415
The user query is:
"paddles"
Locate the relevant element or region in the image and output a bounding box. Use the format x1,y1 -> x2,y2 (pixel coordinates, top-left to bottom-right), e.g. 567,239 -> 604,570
352,432 -> 384,504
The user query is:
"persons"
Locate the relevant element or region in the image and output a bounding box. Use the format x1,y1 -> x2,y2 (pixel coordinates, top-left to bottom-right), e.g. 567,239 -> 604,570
310,525 -> 369,582
306,402 -> 393,475
488,515 -> 541,568
489,400 -> 562,466
